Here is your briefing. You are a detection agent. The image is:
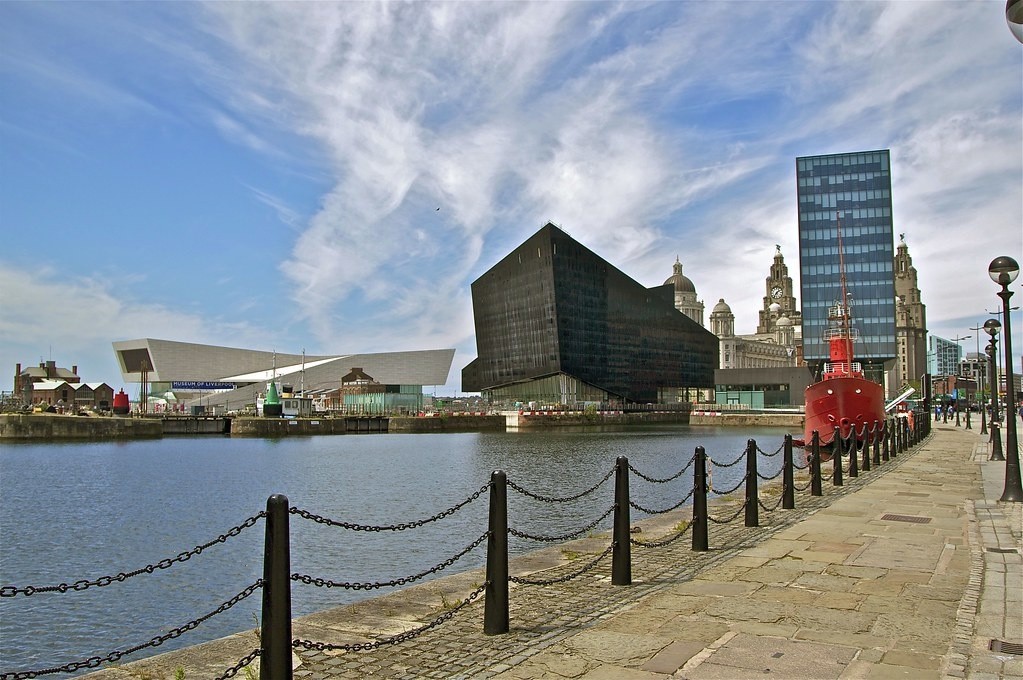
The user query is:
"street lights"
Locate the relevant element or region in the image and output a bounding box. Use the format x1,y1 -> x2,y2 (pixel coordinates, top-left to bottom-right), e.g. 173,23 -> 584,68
988,256 -> 1023,502
989,304 -> 1020,423
969,320 -> 984,414
199,390 -> 201,405
927,334 -> 972,379
954,373 -> 961,426
983,319 -> 1006,461
977,357 -> 988,434
962,367 -> 972,429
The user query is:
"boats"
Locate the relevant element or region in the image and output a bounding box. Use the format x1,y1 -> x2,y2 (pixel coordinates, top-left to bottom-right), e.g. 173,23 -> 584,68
804,211 -> 887,451
793,439 -> 804,447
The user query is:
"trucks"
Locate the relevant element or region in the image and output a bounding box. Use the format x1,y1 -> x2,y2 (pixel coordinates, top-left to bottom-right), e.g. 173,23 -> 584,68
989,399 -> 1007,408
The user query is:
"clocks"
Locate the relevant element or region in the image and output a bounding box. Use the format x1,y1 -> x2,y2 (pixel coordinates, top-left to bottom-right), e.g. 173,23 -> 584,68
770,286 -> 783,299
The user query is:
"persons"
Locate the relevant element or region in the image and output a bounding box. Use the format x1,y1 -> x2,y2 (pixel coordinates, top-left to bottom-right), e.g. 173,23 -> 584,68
937,404 -> 1023,422
